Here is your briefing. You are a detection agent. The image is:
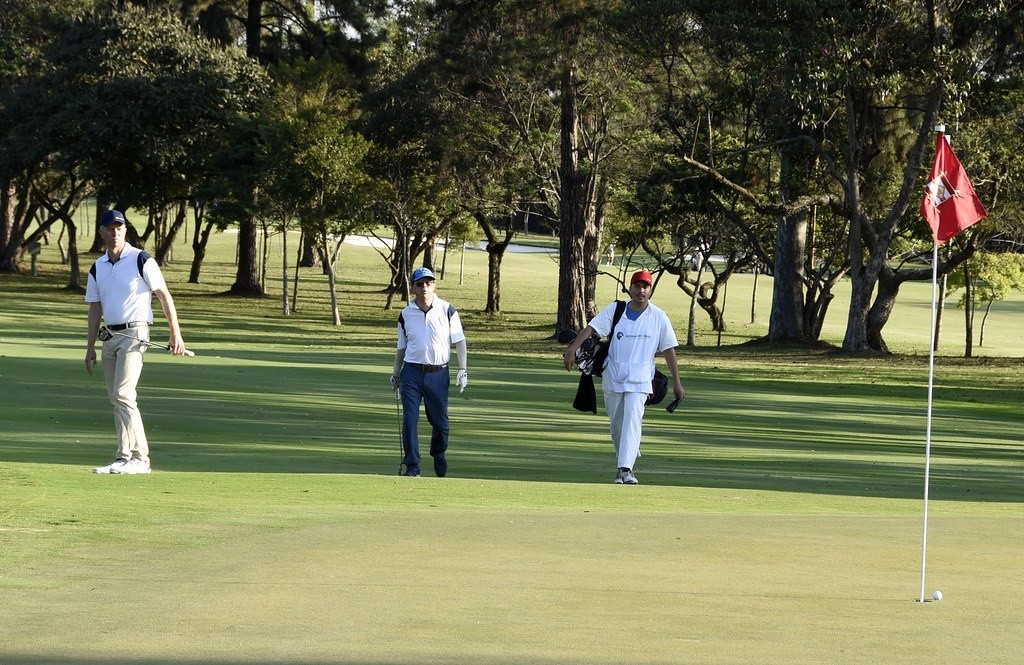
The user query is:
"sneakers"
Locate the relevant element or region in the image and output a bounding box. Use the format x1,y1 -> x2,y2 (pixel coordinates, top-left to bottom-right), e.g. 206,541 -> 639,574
620,471 -> 638,483
110,457 -> 152,473
93,459 -> 127,474
430,451 -> 447,477
404,467 -> 421,477
615,470 -> 624,484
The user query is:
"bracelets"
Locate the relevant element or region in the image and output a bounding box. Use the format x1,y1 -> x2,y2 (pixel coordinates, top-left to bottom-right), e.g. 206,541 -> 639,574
458,368 -> 467,372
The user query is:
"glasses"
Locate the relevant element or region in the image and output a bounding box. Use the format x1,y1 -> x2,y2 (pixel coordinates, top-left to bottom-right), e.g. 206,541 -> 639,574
414,280 -> 435,288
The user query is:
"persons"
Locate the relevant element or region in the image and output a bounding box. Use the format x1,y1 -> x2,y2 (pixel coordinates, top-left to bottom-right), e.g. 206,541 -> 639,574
85,211 -> 184,475
390,268 -> 468,475
565,272 -> 685,484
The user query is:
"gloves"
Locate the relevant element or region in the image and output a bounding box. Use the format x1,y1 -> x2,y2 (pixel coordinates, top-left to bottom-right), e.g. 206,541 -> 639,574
390,375 -> 401,391
456,370 -> 468,393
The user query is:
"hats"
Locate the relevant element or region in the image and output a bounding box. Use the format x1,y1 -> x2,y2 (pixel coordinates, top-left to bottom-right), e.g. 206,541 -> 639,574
98,209 -> 123,226
411,267 -> 436,283
630,271 -> 653,287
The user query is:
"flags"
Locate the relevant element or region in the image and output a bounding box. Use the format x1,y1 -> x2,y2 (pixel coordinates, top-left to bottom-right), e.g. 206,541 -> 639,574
921,134 -> 988,245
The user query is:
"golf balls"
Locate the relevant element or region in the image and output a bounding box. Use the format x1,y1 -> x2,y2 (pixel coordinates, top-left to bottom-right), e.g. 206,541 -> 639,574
932,591 -> 942,600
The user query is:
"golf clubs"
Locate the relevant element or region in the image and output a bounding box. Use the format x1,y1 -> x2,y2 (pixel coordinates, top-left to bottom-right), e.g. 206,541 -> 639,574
395,385 -> 405,477
97,325 -> 195,357
574,349 -> 595,376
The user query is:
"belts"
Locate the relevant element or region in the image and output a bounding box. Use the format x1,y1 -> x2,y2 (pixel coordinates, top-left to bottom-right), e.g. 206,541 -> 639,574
107,320 -> 148,332
404,362 -> 449,373
